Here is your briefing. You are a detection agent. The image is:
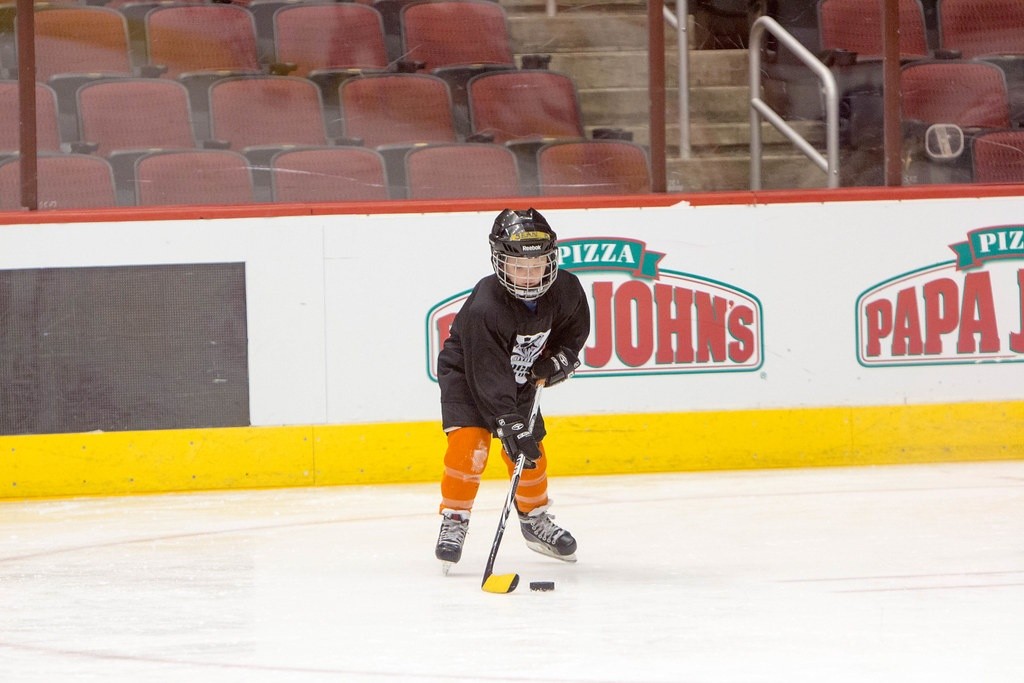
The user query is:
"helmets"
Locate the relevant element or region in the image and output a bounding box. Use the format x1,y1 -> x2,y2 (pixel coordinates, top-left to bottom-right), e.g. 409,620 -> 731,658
489,207 -> 558,300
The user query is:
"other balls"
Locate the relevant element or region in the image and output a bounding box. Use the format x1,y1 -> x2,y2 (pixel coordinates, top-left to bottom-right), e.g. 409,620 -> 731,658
529,581 -> 555,591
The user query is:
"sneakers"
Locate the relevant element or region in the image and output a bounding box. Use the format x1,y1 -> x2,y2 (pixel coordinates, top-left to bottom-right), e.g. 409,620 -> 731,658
514,500 -> 577,562
435,508 -> 471,575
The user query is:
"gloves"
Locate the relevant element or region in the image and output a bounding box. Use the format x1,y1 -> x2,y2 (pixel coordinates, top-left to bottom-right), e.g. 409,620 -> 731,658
525,347 -> 581,387
490,414 -> 542,469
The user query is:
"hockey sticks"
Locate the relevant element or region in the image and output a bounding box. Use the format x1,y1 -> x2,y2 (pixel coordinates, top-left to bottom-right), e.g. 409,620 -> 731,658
480,378 -> 546,594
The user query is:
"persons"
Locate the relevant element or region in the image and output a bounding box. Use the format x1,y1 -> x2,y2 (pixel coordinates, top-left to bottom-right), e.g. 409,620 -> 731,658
436,207 -> 591,579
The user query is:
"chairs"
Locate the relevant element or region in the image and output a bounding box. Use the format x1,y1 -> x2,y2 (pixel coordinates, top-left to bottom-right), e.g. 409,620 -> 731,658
816,0 -> 1024,186
0,0 -> 652,210
